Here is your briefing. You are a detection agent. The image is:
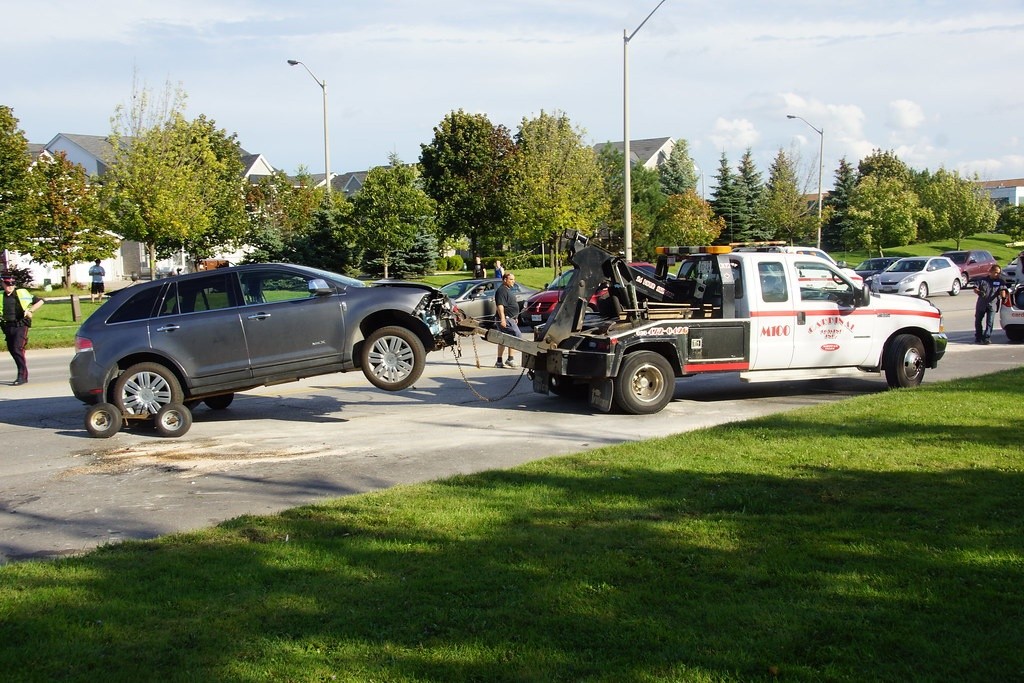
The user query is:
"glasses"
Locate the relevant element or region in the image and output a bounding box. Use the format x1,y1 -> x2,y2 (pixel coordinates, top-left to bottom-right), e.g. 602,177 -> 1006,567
3,281 -> 17,286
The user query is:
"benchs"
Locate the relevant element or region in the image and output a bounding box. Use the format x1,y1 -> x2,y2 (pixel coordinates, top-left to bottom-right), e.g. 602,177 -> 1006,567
735,275 -> 783,301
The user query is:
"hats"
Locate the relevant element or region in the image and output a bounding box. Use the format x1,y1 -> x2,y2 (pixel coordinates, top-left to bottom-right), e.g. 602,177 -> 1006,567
1,273 -> 18,283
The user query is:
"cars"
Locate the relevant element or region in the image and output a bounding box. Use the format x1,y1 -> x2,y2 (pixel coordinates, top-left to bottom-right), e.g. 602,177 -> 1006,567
853,257 -> 911,289
66,262 -> 460,421
939,250 -> 1002,291
870,256 -> 964,299
1000,250 -> 1024,341
519,261 -> 659,326
434,277 -> 543,328
728,241 -> 864,293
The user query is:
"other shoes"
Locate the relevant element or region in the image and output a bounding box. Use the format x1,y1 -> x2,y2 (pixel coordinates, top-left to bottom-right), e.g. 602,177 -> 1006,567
13,379 -> 28,386
505,359 -> 519,367
495,362 -> 504,368
975,337 -> 981,343
982,337 -> 992,344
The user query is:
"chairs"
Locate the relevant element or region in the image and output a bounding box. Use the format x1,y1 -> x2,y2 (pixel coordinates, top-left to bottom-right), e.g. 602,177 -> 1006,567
160,302 -> 183,316
241,278 -> 260,305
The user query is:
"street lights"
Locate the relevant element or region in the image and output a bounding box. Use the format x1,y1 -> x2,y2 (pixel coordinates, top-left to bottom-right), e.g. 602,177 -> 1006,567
287,58 -> 331,193
787,115 -> 825,246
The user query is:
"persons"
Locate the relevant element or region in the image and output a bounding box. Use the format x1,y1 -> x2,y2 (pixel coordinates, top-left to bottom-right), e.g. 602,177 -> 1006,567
494,273 -> 523,367
89,258 -> 105,303
0,271 -> 46,385
928,263 -> 936,270
473,255 -> 505,297
974,265 -> 1009,344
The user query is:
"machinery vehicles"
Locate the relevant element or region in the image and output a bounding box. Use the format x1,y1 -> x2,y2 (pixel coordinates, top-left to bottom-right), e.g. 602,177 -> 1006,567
441,240 -> 951,416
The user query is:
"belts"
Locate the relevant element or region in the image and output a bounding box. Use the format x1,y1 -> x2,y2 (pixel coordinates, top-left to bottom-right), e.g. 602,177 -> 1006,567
4,321 -> 25,327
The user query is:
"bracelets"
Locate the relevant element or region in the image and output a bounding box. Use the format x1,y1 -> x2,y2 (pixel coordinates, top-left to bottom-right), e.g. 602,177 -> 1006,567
29,309 -> 35,313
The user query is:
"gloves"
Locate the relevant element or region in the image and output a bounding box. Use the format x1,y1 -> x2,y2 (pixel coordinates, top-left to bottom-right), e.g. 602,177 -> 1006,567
978,291 -> 986,298
